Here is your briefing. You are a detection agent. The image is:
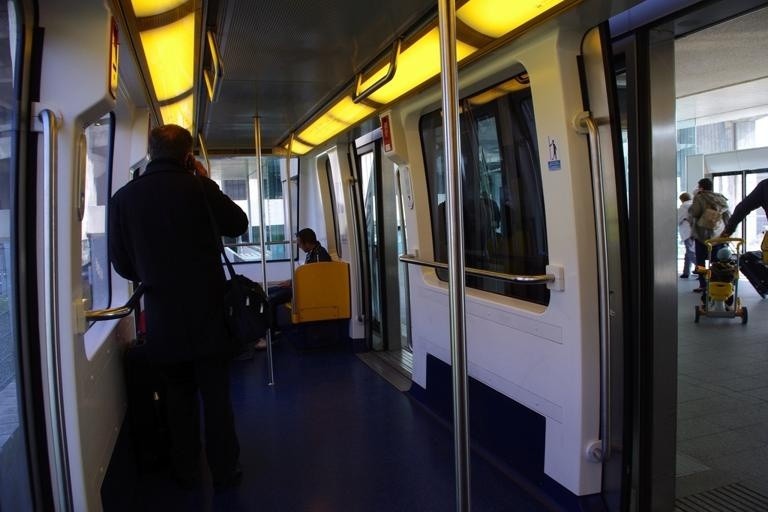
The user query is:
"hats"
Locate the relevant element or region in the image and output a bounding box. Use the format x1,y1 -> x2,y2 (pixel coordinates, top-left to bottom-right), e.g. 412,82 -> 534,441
717,247 -> 732,260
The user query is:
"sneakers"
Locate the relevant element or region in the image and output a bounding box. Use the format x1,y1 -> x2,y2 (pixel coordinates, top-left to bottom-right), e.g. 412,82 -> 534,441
693,288 -> 706,292
255,337 -> 274,348
680,273 -> 688,278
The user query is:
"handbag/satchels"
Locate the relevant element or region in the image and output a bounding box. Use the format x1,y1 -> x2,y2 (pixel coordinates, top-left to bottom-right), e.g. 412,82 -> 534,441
224,275 -> 269,349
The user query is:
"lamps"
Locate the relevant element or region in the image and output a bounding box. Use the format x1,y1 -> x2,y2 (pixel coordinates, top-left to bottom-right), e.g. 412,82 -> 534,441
276,1 -> 582,158
119,2 -> 204,145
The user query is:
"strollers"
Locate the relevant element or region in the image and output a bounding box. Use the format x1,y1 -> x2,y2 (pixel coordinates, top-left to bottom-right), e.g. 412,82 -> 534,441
694,237 -> 748,325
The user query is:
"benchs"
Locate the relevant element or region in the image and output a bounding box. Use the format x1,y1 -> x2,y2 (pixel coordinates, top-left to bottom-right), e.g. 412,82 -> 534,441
284,261 -> 352,324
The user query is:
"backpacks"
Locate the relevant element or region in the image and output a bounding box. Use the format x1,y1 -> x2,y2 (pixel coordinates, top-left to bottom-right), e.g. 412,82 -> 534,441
696,194 -> 729,244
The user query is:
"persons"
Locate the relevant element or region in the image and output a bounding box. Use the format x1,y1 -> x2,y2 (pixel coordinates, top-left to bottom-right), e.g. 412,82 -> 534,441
255,228 -> 332,349
677,178 -> 768,312
107,124 -> 248,499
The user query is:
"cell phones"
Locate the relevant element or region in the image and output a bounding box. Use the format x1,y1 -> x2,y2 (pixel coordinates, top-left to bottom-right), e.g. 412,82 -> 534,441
185,152 -> 195,174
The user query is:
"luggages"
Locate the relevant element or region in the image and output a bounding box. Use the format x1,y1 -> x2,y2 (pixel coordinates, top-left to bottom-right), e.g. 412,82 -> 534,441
726,238 -> 768,299
